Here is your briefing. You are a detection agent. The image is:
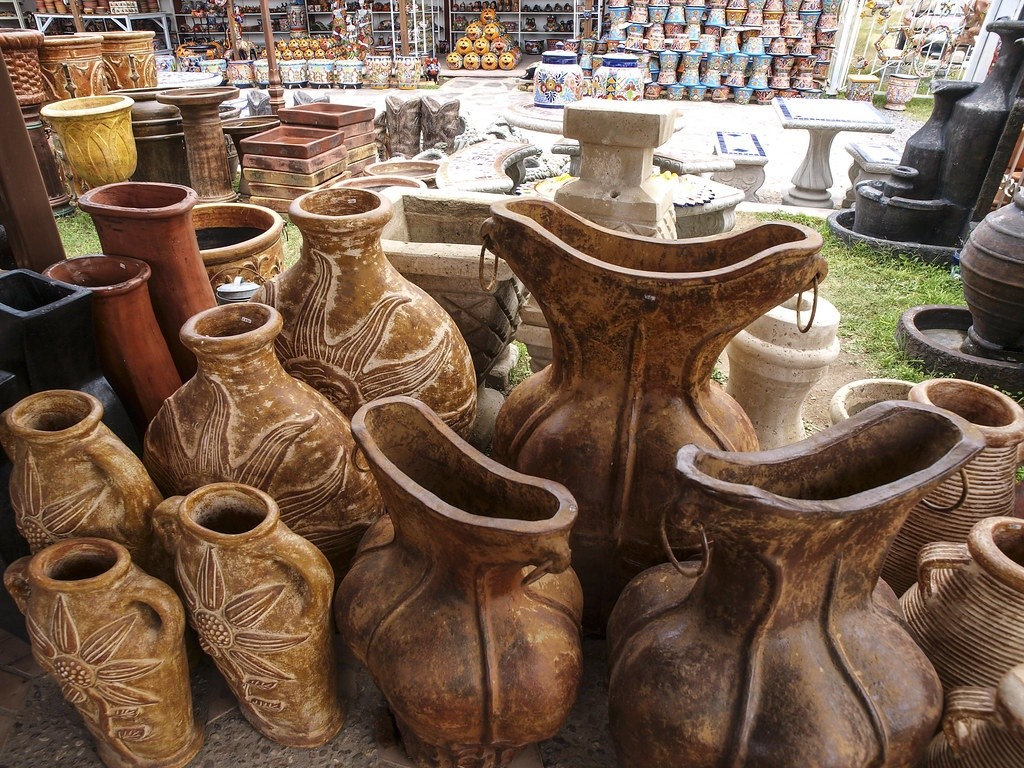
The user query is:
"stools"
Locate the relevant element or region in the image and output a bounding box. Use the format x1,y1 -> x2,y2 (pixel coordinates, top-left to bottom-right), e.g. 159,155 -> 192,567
848,74 -> 879,105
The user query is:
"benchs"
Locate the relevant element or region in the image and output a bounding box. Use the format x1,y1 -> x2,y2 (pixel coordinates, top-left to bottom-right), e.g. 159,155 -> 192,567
710,130 -> 770,204
675,180 -> 746,242
839,143 -> 906,207
436,136 -> 539,193
548,137 -> 736,176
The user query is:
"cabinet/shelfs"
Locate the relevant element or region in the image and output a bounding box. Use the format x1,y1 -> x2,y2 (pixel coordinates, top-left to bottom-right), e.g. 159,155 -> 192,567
0,0 -> 604,63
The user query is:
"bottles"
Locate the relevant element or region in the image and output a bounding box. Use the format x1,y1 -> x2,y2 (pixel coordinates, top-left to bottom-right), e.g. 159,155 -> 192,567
0,177 -> 1023,767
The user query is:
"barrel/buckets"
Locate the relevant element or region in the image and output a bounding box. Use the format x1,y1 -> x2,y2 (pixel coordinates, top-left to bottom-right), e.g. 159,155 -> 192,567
0,26 -> 159,221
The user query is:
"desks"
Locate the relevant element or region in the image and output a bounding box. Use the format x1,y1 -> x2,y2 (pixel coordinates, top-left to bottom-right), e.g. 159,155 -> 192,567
503,97 -> 690,171
771,95 -> 897,207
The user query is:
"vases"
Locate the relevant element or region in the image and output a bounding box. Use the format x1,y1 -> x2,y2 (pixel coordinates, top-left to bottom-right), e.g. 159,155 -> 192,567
0,90 -> 1024,768
